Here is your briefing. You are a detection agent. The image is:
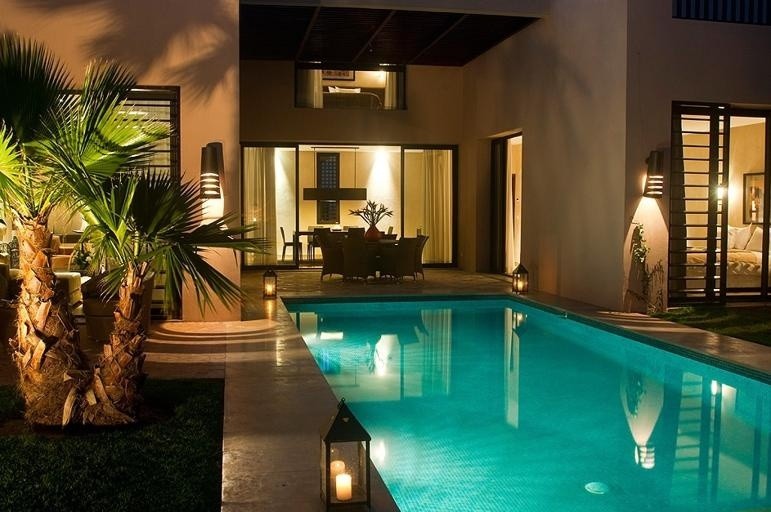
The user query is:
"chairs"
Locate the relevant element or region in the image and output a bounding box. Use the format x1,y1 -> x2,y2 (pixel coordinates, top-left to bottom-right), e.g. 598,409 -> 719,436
278,223 -> 430,286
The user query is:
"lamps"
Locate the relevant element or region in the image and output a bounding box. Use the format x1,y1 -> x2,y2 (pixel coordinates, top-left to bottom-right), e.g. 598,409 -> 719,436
642,148 -> 665,200
198,145 -> 223,203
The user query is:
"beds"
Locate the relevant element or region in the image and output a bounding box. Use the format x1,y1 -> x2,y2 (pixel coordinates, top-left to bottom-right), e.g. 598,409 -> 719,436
686,233 -> 763,288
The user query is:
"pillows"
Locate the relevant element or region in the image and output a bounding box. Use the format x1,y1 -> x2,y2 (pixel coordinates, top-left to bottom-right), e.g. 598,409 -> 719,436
708,224 -> 762,251
327,84 -> 363,93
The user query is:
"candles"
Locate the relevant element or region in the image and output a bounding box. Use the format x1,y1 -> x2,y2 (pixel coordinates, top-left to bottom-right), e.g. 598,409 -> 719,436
331,459 -> 346,479
335,474 -> 352,501
518,280 -> 523,291
266,283 -> 273,296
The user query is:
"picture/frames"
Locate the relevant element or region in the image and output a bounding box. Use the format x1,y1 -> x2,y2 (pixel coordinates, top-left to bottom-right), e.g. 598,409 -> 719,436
743,170 -> 763,225
322,67 -> 355,81
